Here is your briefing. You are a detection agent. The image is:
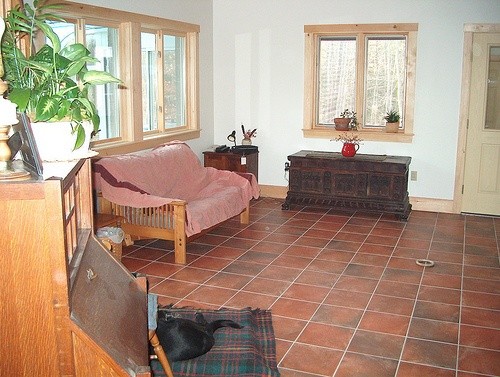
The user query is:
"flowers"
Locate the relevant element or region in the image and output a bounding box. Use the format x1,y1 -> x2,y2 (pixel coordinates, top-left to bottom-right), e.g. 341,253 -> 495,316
330,132 -> 364,145
242,124 -> 257,139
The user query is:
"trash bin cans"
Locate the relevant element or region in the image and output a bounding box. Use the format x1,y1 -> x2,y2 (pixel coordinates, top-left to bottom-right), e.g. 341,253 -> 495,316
96,235 -> 123,262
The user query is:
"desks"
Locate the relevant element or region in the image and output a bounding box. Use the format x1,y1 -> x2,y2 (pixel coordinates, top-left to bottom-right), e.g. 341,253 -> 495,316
281,150 -> 414,222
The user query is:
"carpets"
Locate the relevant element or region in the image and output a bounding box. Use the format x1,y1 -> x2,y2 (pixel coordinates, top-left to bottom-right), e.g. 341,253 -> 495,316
149,304 -> 281,377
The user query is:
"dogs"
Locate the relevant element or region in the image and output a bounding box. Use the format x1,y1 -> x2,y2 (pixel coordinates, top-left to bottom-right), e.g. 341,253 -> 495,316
147,309 -> 245,364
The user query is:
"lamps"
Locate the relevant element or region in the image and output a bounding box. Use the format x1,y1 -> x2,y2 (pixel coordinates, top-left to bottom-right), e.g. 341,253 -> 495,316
227,130 -> 240,154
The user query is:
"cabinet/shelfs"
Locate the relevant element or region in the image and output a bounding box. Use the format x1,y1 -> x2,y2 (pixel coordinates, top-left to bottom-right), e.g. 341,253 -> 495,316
0,157 -> 152,377
201,146 -> 258,185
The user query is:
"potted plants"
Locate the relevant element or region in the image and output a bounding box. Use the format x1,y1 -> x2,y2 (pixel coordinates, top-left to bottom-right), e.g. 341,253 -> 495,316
0,0 -> 94,163
383,110 -> 400,134
333,109 -> 360,131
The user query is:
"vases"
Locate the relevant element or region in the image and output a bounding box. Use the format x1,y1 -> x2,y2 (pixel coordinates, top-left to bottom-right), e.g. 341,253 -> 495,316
242,138 -> 253,146
341,143 -> 360,157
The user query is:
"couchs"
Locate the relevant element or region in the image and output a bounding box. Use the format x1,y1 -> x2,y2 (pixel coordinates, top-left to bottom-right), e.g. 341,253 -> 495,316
90,139 -> 260,266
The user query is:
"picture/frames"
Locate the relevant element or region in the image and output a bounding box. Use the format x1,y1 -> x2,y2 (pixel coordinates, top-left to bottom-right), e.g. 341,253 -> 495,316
17,113 -> 44,178
